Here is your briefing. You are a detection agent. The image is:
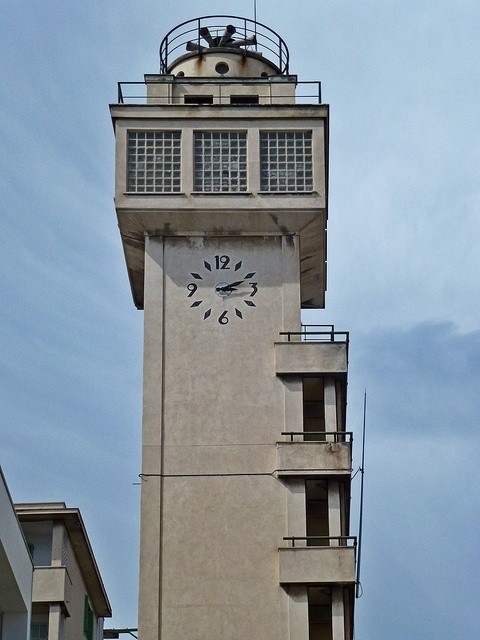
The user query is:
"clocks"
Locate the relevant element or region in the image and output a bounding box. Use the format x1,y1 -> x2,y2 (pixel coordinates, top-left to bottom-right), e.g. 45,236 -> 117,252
186,255 -> 259,327
